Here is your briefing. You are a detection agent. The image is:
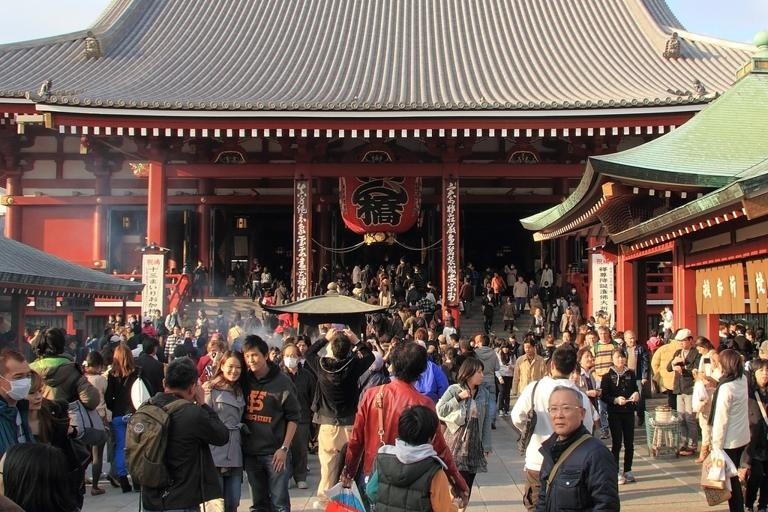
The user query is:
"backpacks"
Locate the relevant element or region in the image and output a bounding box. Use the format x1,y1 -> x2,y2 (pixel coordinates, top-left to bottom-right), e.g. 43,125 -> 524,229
124,390 -> 193,489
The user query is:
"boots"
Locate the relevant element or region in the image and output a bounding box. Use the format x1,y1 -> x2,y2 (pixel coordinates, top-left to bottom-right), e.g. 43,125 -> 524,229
694,445 -> 710,463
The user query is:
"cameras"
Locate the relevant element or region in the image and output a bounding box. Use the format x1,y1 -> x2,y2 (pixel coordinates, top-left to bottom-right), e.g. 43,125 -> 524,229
336,330 -> 345,335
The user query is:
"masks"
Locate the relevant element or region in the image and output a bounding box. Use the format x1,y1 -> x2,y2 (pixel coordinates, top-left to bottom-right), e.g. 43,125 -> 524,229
283,356 -> 299,369
0,374 -> 32,401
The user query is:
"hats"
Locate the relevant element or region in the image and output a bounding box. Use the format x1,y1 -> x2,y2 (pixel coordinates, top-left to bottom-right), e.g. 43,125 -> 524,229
110,335 -> 121,343
143,316 -> 151,323
674,328 -> 692,341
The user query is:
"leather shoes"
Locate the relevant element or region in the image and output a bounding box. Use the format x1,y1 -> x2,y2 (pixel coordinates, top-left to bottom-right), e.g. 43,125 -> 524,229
84,474 -> 139,496
637,414 -> 645,426
308,438 -> 318,454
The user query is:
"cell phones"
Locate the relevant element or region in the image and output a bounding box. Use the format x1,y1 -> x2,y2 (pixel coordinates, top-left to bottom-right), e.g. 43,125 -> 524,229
625,398 -> 633,401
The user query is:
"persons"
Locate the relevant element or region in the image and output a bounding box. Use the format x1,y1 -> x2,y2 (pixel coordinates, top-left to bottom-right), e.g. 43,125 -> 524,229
0,257 -> 767,512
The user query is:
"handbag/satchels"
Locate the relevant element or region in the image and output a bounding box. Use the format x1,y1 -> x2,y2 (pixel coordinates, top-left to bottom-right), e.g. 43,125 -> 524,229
65,377 -> 107,446
515,379 -> 540,459
700,446 -> 738,506
130,369 -> 152,413
321,480 -> 367,512
442,389 -> 483,460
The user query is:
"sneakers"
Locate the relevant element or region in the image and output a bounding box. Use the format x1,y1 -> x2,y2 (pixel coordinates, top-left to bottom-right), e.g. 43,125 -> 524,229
490,423 -> 496,429
297,480 -> 308,489
599,427 -> 610,439
617,469 -> 636,485
498,408 -> 510,417
675,449 -> 695,456
746,507 -> 767,512
